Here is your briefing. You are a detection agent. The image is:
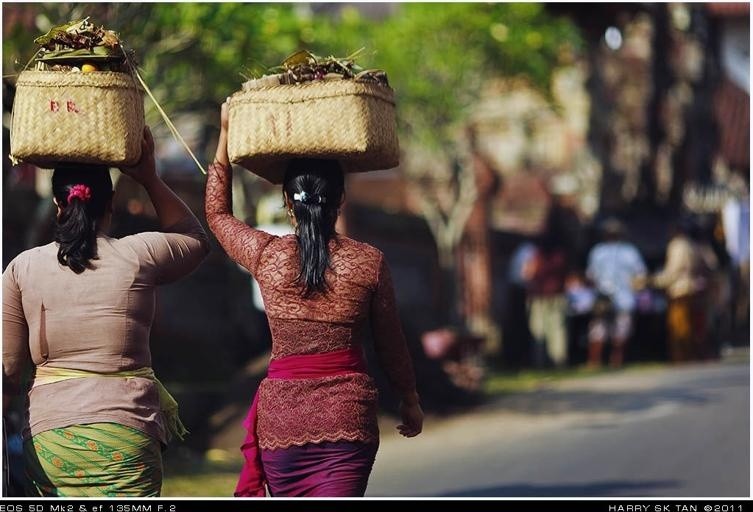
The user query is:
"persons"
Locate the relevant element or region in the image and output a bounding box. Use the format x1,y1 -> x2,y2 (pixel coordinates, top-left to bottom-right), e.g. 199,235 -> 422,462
708,156 -> 748,346
506,221 -> 596,368
575,211 -> 652,369
1,124 -> 210,499
202,91 -> 429,499
628,220 -> 721,371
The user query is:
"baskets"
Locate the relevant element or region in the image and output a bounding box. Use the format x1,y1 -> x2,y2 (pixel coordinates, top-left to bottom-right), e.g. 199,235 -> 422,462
227,80 -> 400,184
10,71 -> 145,168
